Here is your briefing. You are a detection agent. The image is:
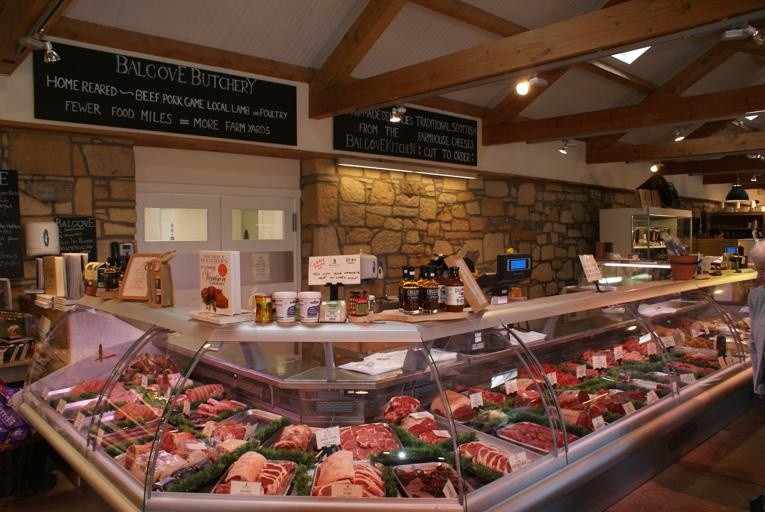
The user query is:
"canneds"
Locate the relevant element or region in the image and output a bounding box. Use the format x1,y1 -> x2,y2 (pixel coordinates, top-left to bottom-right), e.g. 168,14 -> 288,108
255,294 -> 272,323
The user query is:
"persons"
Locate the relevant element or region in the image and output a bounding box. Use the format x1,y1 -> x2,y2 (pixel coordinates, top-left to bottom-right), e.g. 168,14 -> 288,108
710,229 -> 725,239
747,241 -> 765,512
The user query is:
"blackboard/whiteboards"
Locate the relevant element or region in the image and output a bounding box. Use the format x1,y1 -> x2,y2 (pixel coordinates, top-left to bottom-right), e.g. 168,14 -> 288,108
0,170 -> 24,278
55,217 -> 97,263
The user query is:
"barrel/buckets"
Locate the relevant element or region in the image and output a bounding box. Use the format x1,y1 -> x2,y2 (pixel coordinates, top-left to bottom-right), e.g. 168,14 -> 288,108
670,255 -> 702,280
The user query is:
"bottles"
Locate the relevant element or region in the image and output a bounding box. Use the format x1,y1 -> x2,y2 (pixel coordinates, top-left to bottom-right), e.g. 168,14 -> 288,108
398,265 -> 464,315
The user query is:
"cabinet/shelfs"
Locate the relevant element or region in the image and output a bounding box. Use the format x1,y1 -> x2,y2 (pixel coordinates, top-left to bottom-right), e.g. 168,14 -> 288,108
703,209 -> 764,239
0,277 -> 80,503
599,207 -> 692,286
7,279 -> 764,510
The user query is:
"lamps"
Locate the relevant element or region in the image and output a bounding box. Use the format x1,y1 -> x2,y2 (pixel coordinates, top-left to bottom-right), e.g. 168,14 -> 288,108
390,104 -> 401,122
725,183 -> 750,200
517,78 -> 532,95
650,162 -> 665,173
558,139 -> 570,156
744,25 -> 759,37
673,124 -> 685,142
41,36 -> 61,64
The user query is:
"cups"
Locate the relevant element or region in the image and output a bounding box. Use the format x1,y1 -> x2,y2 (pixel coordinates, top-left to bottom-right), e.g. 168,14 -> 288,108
254,292 -> 321,323
